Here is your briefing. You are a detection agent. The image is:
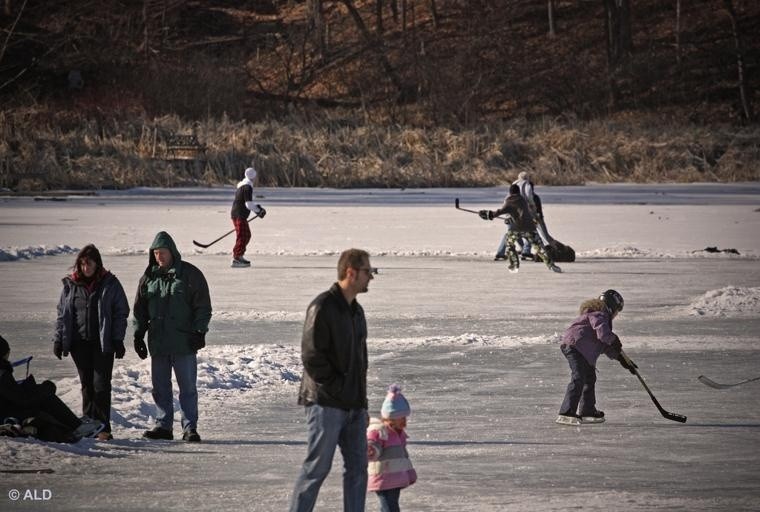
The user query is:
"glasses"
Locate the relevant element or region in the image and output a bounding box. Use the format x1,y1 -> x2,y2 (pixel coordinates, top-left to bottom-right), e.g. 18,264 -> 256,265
358,267 -> 378,275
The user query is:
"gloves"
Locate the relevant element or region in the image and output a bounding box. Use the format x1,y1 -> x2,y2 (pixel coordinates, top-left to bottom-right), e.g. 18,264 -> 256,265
620,356 -> 638,371
611,335 -> 623,351
478,210 -> 493,220
133,338 -> 148,360
253,204 -> 266,218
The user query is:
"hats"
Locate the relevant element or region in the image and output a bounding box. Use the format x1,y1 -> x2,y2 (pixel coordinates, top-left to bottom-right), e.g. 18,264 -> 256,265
245,167 -> 257,180
381,382 -> 410,420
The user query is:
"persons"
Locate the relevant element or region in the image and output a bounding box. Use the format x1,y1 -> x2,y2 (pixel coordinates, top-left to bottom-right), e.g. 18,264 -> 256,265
479,184 -> 555,271
557,290 -> 638,418
53,243 -> 131,442
366,382 -> 417,512
230,167 -> 266,265
287,248 -> 374,511
132,233 -> 213,442
517,181 -> 544,254
495,171 -> 537,259
0,335 -> 102,442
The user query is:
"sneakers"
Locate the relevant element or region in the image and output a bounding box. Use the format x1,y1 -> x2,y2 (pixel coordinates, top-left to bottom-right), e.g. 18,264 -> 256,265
508,261 -> 518,269
184,433 -> 200,441
72,419 -> 101,438
98,431 -> 114,442
559,412 -> 582,421
577,411 -> 605,421
142,426 -> 173,440
232,256 -> 250,266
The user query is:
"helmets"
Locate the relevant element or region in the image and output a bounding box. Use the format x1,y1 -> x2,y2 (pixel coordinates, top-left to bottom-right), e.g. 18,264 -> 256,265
598,289 -> 624,314
